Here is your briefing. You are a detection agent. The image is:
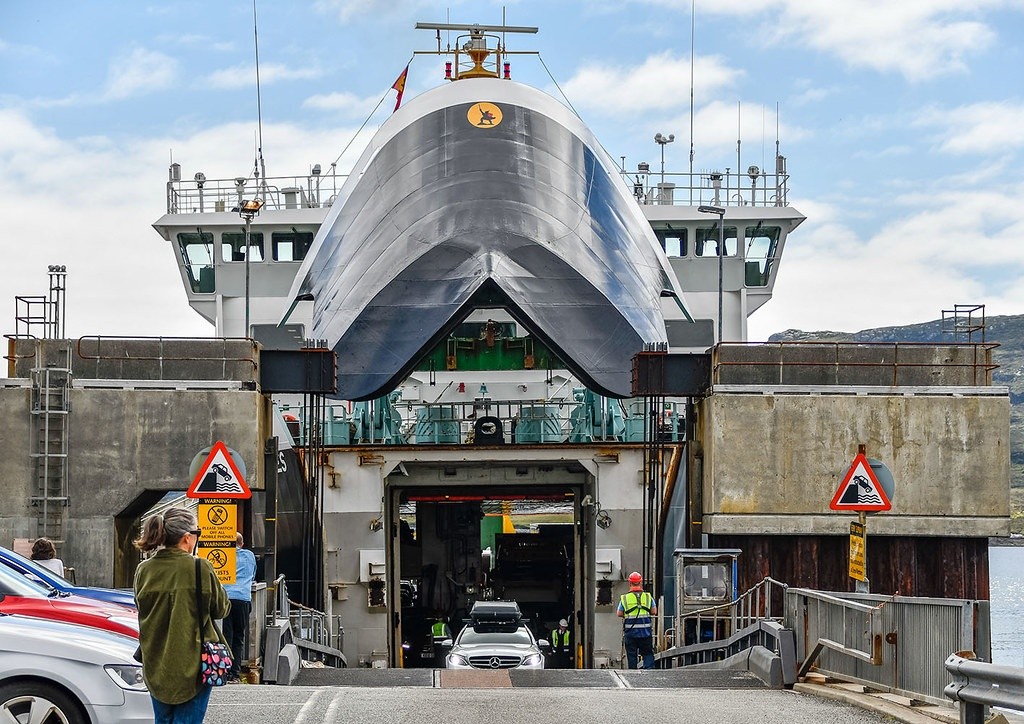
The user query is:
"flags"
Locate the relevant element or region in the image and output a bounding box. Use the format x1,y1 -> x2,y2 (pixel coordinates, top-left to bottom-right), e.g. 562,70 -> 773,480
390,64 -> 409,113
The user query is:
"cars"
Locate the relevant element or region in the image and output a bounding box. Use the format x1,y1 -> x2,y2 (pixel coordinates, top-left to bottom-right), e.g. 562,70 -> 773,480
441,599 -> 551,669
0,545 -> 159,724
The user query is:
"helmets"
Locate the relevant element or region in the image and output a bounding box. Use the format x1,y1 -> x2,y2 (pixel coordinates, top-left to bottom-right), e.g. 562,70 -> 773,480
628,572 -> 643,583
559,619 -> 568,626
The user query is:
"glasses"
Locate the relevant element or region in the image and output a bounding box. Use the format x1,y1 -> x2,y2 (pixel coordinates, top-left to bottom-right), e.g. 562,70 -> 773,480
189,529 -> 201,537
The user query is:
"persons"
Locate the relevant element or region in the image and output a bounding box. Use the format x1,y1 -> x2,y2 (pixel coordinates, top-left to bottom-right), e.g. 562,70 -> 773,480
222,532 -> 258,681
617,572 -> 657,669
24,538 -> 64,588
547,619 -> 574,669
432,615 -> 453,668
133,507 -> 232,724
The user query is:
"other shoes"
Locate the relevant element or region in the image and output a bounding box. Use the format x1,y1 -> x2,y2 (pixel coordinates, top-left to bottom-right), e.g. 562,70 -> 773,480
227,678 -> 241,684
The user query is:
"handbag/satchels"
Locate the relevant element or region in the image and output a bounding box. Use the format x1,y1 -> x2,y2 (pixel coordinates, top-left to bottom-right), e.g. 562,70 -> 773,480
201,641 -> 232,686
